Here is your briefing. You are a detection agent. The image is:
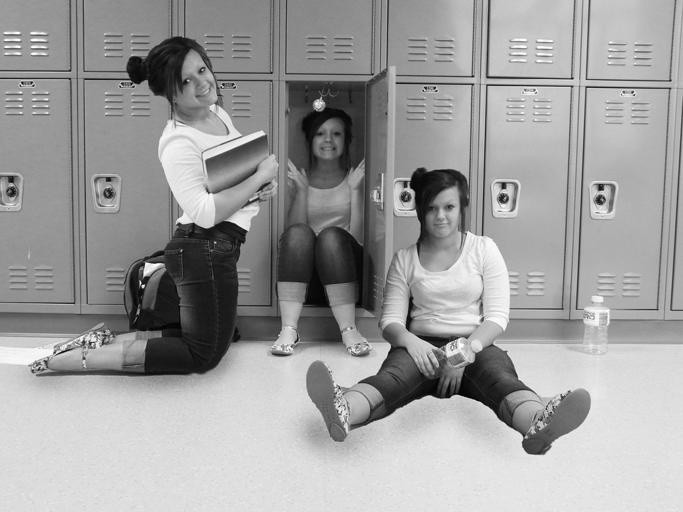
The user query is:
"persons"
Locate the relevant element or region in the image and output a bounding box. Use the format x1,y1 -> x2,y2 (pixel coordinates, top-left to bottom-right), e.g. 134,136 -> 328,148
28,36 -> 280,377
306,169 -> 591,455
270,107 -> 372,358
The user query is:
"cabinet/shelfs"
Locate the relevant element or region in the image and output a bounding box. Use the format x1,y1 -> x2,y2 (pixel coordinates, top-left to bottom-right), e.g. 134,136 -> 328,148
665,95 -> 683,323
569,80 -> 677,322
0,0 -> 78,76
277,66 -> 398,318
476,83 -> 578,321
77,78 -> 178,316
76,0 -> 177,79
393,81 -> 479,259
177,1 -> 280,80
379,0 -> 483,81
178,80 -> 279,318
280,1 -> 382,75
483,1 -> 584,83
580,0 -> 683,81
0,76 -> 77,317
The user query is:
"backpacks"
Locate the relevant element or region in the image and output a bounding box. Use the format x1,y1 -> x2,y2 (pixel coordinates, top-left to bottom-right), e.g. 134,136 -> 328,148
123,252 -> 240,342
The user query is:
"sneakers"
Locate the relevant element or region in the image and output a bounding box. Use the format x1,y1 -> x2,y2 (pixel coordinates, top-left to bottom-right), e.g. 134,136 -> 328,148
305,359 -> 350,442
53,320 -> 115,353
27,329 -> 104,374
271,326 -> 300,356
521,388 -> 591,455
339,326 -> 371,356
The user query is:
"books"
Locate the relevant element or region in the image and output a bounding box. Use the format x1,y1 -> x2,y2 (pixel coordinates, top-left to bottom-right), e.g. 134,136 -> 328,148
202,130 -> 273,211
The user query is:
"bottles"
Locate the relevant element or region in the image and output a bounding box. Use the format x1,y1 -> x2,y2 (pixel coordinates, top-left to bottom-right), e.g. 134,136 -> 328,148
583,296 -> 609,355
433,337 -> 483,378
